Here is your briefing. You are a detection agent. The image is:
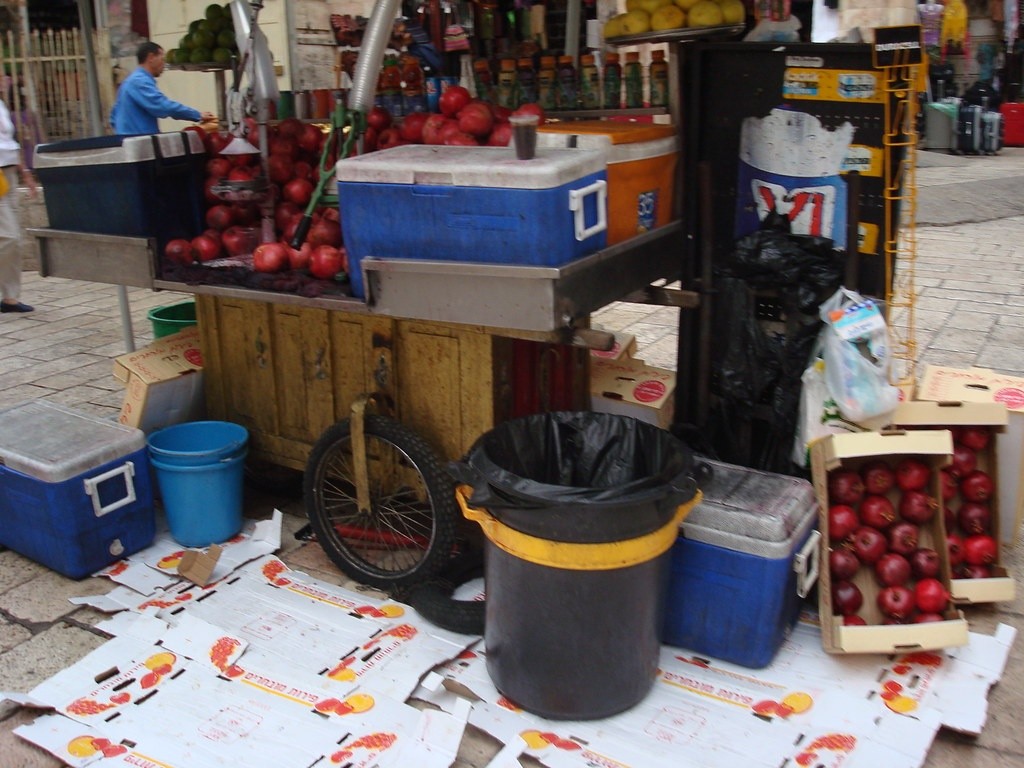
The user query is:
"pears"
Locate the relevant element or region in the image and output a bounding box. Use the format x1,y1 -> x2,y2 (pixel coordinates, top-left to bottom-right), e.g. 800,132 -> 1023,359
603,0 -> 745,39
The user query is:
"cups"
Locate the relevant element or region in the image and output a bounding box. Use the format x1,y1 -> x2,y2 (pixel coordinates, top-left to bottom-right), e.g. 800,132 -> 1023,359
508,115 -> 540,159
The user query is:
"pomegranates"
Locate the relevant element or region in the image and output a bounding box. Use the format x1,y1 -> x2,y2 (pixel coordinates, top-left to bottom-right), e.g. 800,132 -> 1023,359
828,427 -> 997,626
165,87 -> 544,278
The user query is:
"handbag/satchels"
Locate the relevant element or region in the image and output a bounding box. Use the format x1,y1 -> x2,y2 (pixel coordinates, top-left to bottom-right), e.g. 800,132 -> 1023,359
820,289 -> 898,418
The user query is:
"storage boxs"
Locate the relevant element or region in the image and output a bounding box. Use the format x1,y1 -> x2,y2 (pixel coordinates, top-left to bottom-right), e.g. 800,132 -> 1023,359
590,355 -> 678,432
782,66 -> 833,101
591,328 -> 639,362
657,454 -> 822,670
837,144 -> 885,178
336,144 -> 609,299
915,362 -> 1024,547
807,429 -> 969,656
857,221 -> 880,255
507,119 -> 682,248
0,397 -> 155,583
27,217 -> 690,334
32,129 -> 211,243
833,70 -> 885,104
889,401 -> 1017,604
110,324 -> 204,437
830,298 -> 887,344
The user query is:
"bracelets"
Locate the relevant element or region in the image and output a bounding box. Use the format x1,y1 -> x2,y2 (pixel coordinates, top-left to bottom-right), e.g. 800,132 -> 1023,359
20,168 -> 31,173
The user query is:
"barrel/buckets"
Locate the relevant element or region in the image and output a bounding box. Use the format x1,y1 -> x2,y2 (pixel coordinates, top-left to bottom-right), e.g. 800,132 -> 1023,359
147,420 -> 248,466
147,301 -> 197,339
148,452 -> 247,547
454,413 -> 714,720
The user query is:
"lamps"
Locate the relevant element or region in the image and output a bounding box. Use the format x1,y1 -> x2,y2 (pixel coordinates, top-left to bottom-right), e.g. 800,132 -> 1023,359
216,55 -> 262,154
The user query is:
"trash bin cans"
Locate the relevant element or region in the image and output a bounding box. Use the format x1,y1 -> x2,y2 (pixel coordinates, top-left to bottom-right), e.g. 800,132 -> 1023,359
925,102 -> 958,149
456,411 -> 699,722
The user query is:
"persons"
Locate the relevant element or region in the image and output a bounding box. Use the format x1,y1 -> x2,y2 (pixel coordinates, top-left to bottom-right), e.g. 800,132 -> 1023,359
8,82 -> 44,184
0,99 -> 39,313
109,42 -> 218,135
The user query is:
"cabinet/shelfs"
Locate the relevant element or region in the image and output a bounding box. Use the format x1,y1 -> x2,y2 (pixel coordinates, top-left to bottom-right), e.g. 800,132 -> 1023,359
193,288 -> 699,530
678,40 -> 920,482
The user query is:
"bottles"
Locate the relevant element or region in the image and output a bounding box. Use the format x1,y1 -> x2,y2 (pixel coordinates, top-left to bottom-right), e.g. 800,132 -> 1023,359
580,55 -> 600,109
383,59 -> 401,117
602,53 -> 621,108
517,59 -> 537,105
277,88 -> 349,119
473,60 -> 494,101
401,58 -> 426,115
559,56 -> 576,108
536,56 -> 557,108
374,73 -> 383,108
497,60 -> 517,108
624,52 -> 642,107
650,50 -> 668,106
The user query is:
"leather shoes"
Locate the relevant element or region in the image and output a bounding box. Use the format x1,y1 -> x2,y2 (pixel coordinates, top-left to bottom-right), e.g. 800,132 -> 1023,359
0,301 -> 33,313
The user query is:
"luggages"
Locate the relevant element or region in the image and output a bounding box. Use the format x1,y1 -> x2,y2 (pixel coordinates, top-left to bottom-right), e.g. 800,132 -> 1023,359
926,80 -> 1024,156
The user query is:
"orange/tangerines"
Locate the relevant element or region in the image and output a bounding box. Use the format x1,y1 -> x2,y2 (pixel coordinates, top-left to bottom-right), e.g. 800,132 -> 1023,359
164,3 -> 246,64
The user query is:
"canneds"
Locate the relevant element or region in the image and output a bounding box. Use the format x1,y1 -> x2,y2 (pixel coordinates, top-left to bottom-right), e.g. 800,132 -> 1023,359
423,75 -> 458,112
